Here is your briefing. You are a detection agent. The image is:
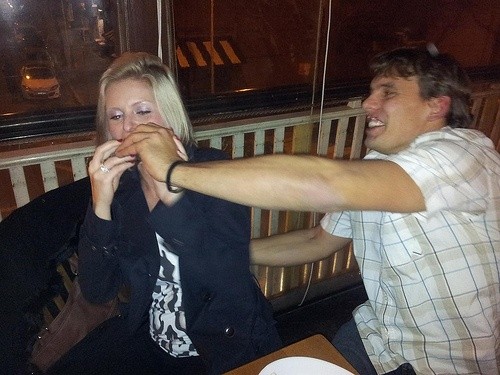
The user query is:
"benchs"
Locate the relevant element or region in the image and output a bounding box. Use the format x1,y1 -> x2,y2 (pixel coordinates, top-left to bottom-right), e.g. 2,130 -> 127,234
273,285 -> 367,347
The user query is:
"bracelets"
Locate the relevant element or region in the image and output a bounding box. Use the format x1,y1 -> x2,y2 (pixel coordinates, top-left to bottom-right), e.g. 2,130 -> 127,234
164,159 -> 185,194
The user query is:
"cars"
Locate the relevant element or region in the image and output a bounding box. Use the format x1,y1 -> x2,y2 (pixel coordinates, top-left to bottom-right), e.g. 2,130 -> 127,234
25,47 -> 54,68
21,65 -> 60,99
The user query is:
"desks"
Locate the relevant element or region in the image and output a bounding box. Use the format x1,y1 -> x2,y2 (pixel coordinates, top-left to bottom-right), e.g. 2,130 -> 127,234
221,334 -> 360,374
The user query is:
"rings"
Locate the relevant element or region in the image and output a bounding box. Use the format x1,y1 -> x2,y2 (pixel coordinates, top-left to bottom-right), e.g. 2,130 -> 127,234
99,163 -> 111,173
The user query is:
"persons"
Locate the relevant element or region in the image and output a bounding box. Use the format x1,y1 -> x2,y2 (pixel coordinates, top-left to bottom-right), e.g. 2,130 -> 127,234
37,52 -> 278,375
112,47 -> 499,374
